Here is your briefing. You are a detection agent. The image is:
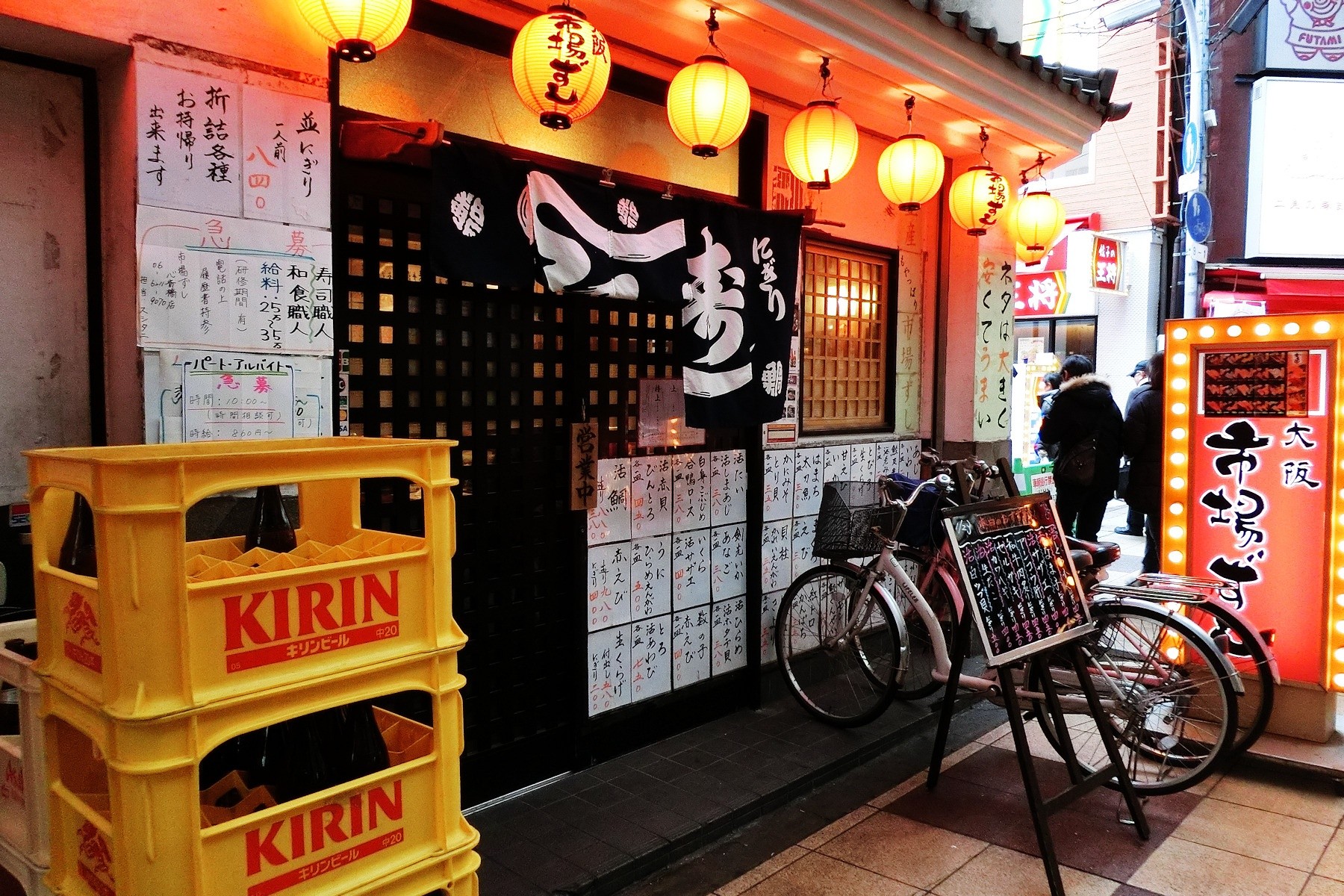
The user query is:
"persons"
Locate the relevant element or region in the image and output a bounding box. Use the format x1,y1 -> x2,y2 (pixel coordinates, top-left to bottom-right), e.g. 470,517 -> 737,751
1114,359 -> 1151,536
1122,351 -> 1165,587
1035,371 -> 1062,458
1038,355 -> 1123,543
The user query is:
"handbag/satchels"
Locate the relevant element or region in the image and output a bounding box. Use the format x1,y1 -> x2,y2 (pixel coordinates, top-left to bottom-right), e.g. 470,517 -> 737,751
1116,460 -> 1131,499
1055,439 -> 1098,487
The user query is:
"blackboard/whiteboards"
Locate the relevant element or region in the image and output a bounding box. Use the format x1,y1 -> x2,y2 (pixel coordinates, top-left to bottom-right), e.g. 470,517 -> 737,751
943,490 -> 1100,666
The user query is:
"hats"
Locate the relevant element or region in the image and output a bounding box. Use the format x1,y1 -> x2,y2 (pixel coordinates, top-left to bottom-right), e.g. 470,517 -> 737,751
1127,359 -> 1149,375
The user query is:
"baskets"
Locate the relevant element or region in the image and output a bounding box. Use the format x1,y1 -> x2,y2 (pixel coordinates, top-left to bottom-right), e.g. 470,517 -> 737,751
812,480 -> 911,557
878,473 -> 957,557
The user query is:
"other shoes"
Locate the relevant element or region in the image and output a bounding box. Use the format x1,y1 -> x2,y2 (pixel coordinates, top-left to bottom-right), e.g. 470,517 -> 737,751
1115,527 -> 1143,536
1126,569 -> 1147,587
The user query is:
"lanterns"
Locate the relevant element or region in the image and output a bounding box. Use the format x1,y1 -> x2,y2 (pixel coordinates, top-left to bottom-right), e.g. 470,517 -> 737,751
267,0 -> 414,65
667,55 -> 751,158
509,4 -> 613,129
1004,191 -> 1067,270
785,99 -> 858,191
878,136 -> 944,212
947,166 -> 1009,236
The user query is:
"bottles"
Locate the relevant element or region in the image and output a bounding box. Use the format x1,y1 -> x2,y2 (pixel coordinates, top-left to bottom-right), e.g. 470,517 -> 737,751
201,699 -> 390,812
244,485 -> 297,568
59,493 -> 97,578
0,639 -> 38,737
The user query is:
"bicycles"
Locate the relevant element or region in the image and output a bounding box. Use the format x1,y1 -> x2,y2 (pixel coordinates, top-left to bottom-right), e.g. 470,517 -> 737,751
774,455 -> 1285,795
